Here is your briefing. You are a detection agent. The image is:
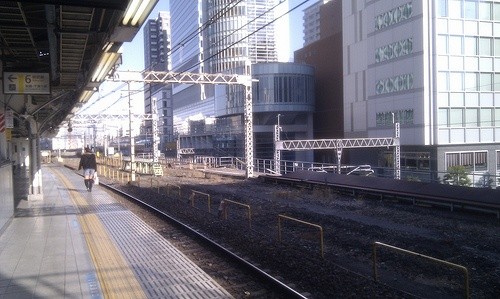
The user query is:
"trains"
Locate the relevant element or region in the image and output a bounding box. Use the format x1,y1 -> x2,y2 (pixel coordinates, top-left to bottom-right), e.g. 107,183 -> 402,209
121,157 -> 164,177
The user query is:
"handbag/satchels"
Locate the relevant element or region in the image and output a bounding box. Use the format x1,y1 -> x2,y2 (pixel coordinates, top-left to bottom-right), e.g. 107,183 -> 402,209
94,171 -> 100,186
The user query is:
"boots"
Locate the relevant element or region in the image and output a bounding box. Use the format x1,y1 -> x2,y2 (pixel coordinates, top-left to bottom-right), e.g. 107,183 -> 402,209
89,179 -> 93,191
84,180 -> 90,191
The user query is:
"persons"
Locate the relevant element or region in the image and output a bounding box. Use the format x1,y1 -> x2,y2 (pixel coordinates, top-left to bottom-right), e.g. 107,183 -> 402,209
78,148 -> 98,192
